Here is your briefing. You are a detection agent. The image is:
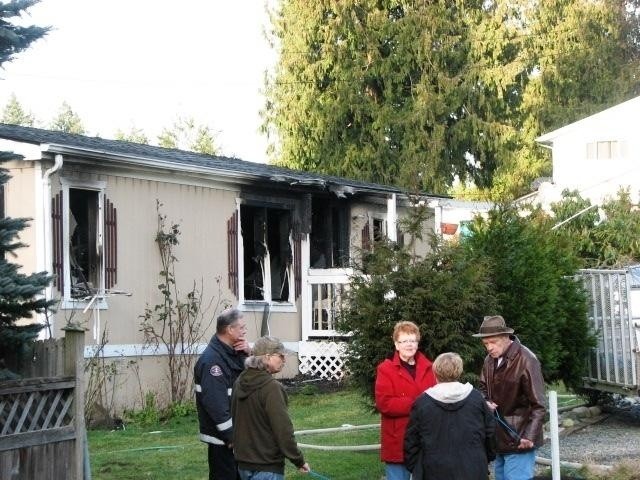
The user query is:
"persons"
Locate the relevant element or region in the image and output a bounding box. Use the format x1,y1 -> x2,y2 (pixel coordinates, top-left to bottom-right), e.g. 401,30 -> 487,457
374,321 -> 437,480
233,335 -> 311,480
472,315 -> 547,480
193,306 -> 252,480
403,352 -> 533,480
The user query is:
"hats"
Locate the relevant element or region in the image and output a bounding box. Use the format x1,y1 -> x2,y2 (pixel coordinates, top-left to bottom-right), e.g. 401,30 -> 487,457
472,315 -> 514,337
251,337 -> 288,356
